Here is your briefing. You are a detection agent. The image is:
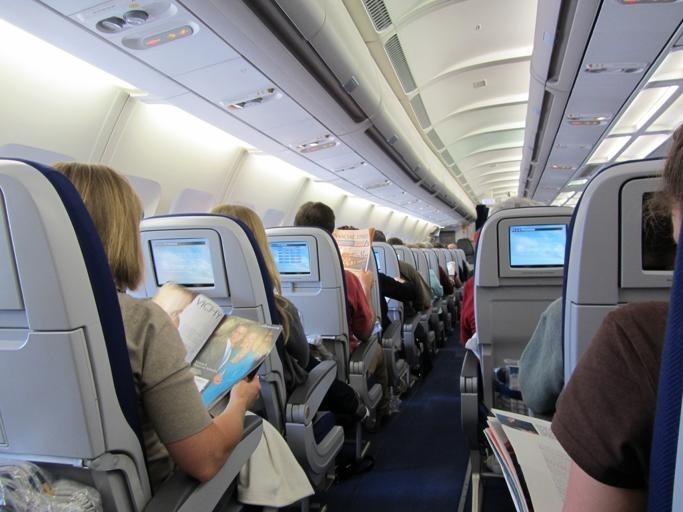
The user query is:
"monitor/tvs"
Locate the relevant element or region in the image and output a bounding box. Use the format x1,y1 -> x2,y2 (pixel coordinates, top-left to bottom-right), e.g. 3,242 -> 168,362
139,228 -> 229,299
267,235 -> 319,281
497,215 -> 572,277
619,175 -> 678,288
373,246 -> 386,274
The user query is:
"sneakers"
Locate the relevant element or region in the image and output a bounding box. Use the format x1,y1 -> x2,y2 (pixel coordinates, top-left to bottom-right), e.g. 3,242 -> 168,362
332,449 -> 374,483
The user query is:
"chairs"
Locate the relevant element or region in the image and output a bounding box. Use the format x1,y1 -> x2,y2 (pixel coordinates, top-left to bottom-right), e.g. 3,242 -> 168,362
263,225 -> 385,463
126,213 -> 343,503
457,155 -> 683,511
1,154 -> 263,510
333,225 -> 473,420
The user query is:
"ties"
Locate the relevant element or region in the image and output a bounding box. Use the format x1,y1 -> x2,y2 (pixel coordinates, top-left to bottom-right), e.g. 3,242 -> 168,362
214,342 -> 233,372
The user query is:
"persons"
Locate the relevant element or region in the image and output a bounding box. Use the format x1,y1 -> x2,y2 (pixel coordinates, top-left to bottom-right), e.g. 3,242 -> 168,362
363,228 -> 475,358
457,195 -> 550,342
204,202 -> 373,479
292,202 -> 399,429
198,331 -> 258,409
553,124 -> 682,512
193,321 -> 249,393
49,159 -> 262,485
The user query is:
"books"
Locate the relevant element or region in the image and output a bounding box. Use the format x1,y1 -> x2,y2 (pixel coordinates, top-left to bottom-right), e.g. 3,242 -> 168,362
150,279 -> 283,415
482,407 -> 571,511
